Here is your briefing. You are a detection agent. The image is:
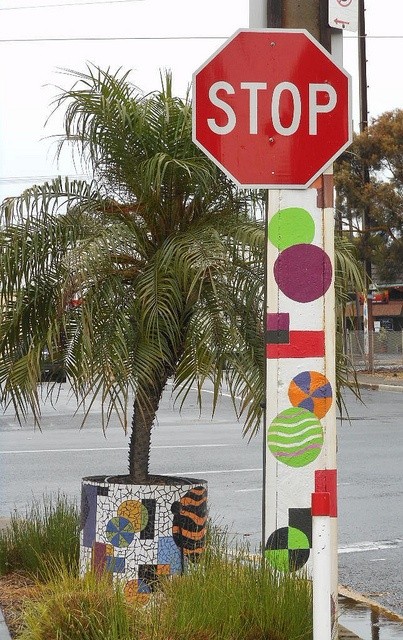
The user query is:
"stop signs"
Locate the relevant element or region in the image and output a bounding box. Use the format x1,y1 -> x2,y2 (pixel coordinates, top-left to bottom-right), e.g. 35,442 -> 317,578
192,28 -> 352,188
